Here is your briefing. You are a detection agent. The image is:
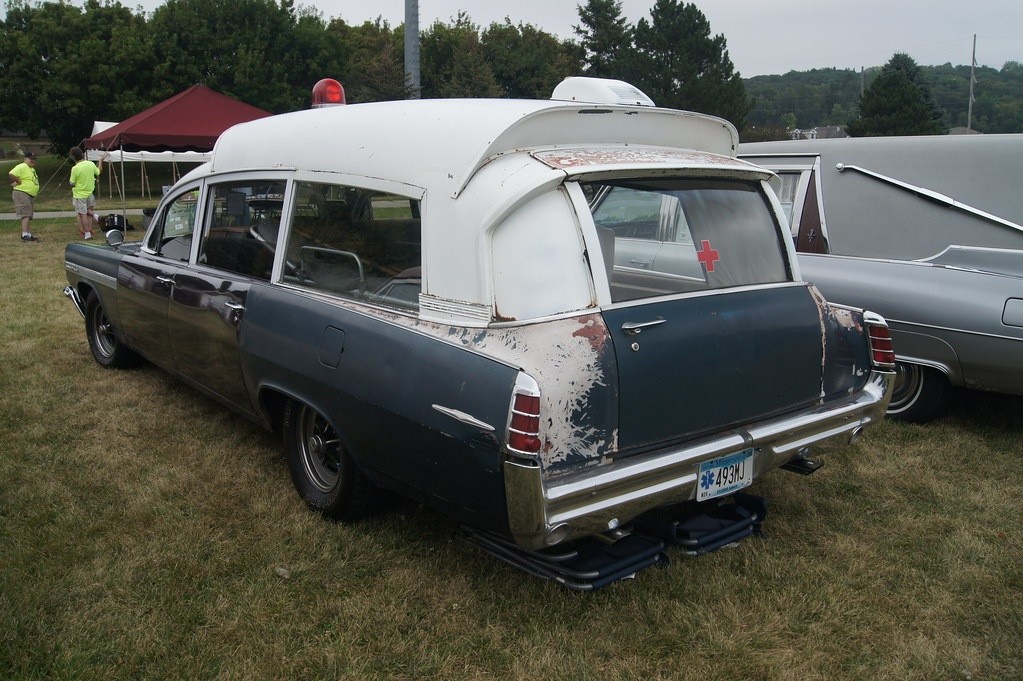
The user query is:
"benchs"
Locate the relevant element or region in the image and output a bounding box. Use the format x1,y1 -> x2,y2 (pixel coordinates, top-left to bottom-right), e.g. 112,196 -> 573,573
297,206 -> 316,217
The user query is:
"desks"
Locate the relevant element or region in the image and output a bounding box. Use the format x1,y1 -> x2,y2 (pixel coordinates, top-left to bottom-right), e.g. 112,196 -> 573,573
178,197 -> 228,223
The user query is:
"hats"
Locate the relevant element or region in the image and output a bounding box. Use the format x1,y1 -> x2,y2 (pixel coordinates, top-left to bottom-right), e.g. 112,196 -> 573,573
25,153 -> 38,159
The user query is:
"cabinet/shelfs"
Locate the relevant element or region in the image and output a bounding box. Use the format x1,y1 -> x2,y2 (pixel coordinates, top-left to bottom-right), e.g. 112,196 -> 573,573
249,199 -> 283,220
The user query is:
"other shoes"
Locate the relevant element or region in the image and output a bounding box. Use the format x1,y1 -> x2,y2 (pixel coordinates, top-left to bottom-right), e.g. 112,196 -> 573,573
93,214 -> 100,224
80,231 -> 93,240
20,235 -> 38,242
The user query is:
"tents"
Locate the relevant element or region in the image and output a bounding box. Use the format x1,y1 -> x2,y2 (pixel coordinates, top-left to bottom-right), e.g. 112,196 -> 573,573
80,82 -> 277,242
82,119 -> 215,204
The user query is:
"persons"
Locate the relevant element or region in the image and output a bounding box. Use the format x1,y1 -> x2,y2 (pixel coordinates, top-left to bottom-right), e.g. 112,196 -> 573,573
69,146 -> 106,241
8,150 -> 41,242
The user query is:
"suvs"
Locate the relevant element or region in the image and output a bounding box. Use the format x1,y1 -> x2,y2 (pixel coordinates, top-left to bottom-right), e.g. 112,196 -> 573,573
735,136 -> 1022,422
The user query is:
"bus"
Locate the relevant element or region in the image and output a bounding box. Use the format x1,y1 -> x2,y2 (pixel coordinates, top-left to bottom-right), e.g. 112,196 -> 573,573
58,73 -> 899,598
